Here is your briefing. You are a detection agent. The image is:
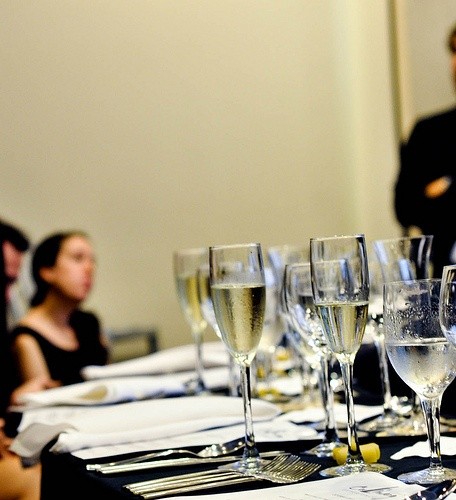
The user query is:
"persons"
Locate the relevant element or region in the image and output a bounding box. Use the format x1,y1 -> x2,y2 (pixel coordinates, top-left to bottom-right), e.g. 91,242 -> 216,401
0,223 -> 108,500
394,28 -> 456,278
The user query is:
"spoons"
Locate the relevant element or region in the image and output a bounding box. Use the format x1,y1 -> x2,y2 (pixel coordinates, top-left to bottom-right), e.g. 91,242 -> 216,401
86,432 -> 254,473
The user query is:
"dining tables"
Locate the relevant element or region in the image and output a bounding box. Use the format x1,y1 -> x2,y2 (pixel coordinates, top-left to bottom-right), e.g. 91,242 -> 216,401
38,335 -> 456,500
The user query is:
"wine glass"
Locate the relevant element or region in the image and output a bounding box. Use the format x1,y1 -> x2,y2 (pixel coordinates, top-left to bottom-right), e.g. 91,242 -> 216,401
172,234 -> 456,484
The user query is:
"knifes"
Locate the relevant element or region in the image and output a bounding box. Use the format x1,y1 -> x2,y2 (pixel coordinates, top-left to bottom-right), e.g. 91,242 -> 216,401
95,451 -> 289,474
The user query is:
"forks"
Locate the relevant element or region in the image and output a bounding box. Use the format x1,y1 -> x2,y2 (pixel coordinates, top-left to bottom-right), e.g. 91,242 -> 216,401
122,453 -> 321,500
